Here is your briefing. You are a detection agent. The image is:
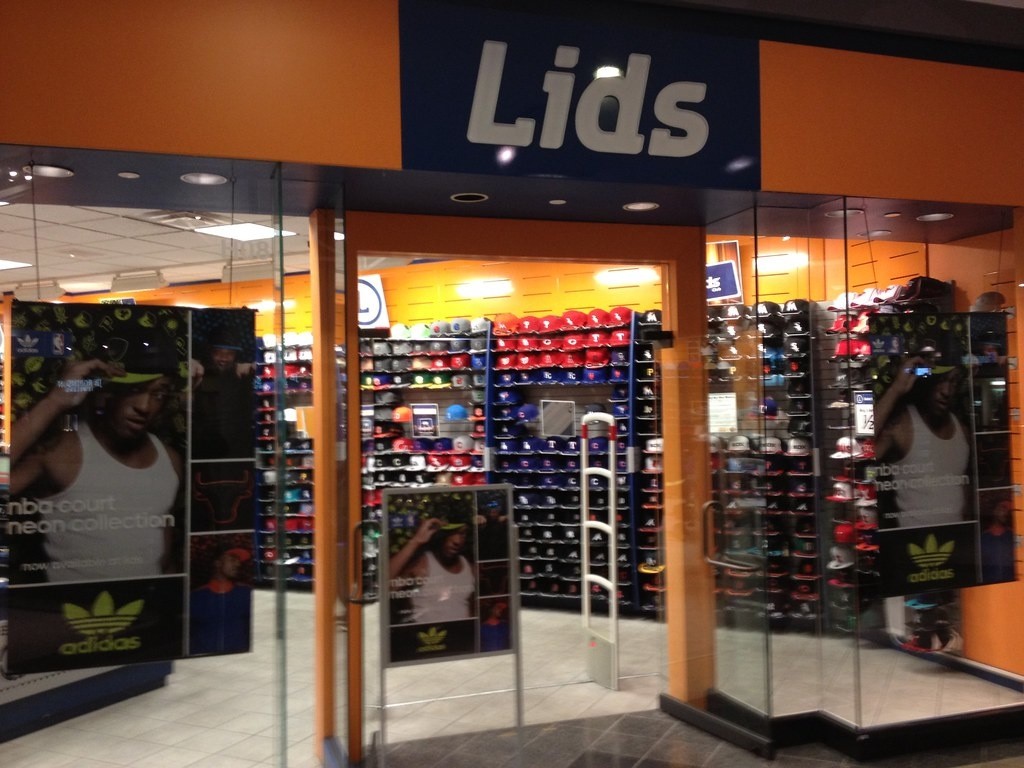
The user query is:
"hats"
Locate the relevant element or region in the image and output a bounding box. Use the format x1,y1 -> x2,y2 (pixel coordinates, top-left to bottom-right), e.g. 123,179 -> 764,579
206,327 -> 243,350
99,341 -> 180,383
993,497 -> 1011,509
434,501 -> 474,529
257,314 -> 489,627
213,542 -> 250,562
705,276 -> 1008,648
490,311 -> 672,623
918,340 -> 967,373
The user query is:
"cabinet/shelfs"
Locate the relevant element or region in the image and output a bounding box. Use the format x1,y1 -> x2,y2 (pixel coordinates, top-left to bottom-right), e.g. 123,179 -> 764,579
255,297 -> 950,630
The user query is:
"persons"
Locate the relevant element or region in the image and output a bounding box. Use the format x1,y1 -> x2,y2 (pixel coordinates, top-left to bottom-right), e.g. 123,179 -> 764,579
192,540 -> 252,653
390,500 -> 476,621
175,329 -> 254,457
11,346 -> 184,577
875,342 -> 972,526
